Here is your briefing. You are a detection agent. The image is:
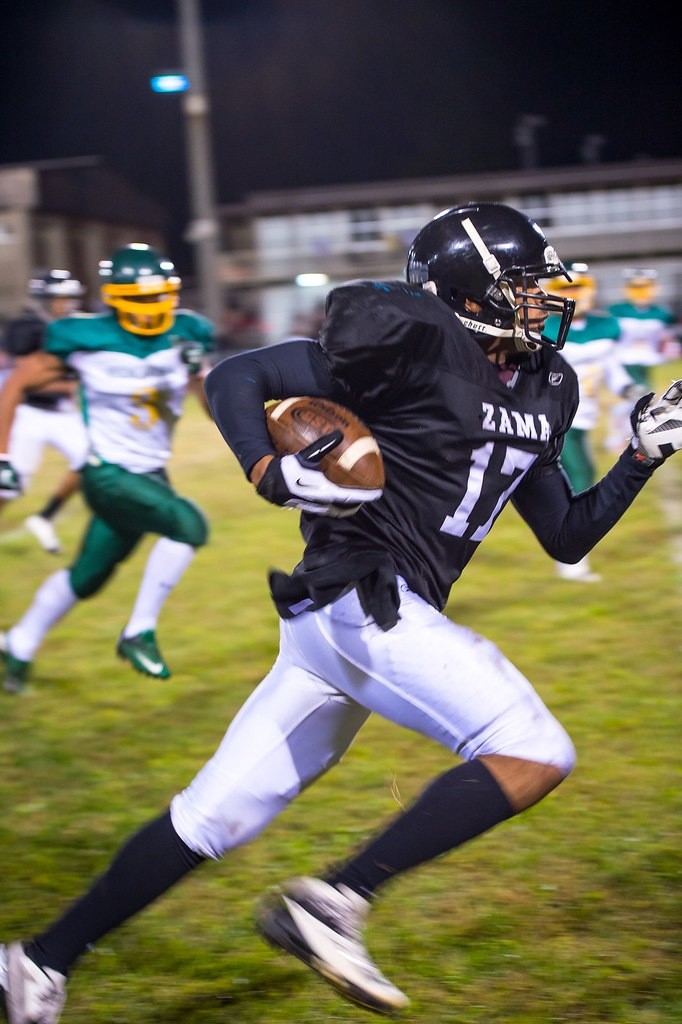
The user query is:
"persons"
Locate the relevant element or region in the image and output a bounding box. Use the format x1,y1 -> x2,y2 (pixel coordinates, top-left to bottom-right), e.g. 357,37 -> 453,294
0,269 -> 86,552
540,264 -> 682,582
0,200 -> 682,1024
0,242 -> 219,688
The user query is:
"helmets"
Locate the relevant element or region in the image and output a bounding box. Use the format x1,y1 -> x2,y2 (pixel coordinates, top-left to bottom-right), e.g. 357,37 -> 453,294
623,269 -> 657,305
538,260 -> 594,316
408,204 -> 573,339
101,242 -> 182,296
27,269 -> 87,296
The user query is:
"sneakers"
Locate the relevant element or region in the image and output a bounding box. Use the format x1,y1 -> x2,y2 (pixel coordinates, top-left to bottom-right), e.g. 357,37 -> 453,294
257,876 -> 410,1016
0,939 -> 68,1024
116,629 -> 170,679
0,633 -> 31,694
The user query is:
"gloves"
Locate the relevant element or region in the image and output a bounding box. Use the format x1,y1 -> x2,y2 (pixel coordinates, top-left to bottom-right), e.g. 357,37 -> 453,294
181,344 -> 205,375
256,430 -> 383,518
629,379 -> 682,459
0,461 -> 21,496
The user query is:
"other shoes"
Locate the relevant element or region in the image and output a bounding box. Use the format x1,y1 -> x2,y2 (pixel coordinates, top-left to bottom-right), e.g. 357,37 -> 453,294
556,558 -> 600,583
24,514 -> 61,553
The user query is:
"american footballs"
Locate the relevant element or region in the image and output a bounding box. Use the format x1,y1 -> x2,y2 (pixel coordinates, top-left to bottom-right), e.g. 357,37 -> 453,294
263,394 -> 385,490
